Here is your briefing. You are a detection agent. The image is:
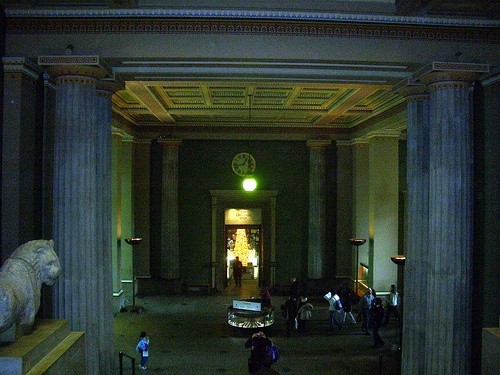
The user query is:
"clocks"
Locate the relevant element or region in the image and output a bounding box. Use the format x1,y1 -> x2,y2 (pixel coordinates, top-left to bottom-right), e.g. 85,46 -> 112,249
232,153 -> 257,177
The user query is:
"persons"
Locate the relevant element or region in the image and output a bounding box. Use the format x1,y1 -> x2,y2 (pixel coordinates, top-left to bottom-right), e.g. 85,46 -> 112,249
136,331 -> 150,370
245,330 -> 273,375
259,282 -> 403,349
232,256 -> 243,287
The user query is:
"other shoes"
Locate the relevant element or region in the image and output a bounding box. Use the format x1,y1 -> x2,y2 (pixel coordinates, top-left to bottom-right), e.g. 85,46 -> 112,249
142,367 -> 147,370
378,342 -> 384,348
361,329 -> 366,332
366,332 -> 371,336
370,344 -> 380,349
138,364 -> 142,369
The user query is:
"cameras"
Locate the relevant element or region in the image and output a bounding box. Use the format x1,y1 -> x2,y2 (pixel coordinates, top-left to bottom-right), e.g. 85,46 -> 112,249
256,333 -> 261,337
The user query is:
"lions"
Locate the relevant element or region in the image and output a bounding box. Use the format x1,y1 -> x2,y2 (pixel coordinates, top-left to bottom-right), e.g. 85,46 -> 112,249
0,239 -> 61,336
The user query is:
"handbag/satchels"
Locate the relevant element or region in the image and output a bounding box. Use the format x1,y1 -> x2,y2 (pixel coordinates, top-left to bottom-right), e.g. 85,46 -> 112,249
265,344 -> 280,362
280,307 -> 289,321
295,318 -> 298,329
333,300 -> 342,310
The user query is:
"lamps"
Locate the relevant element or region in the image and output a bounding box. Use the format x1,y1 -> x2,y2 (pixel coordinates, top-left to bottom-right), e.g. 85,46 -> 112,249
121,238 -> 145,313
390,255 -> 405,266
348,239 -> 366,312
